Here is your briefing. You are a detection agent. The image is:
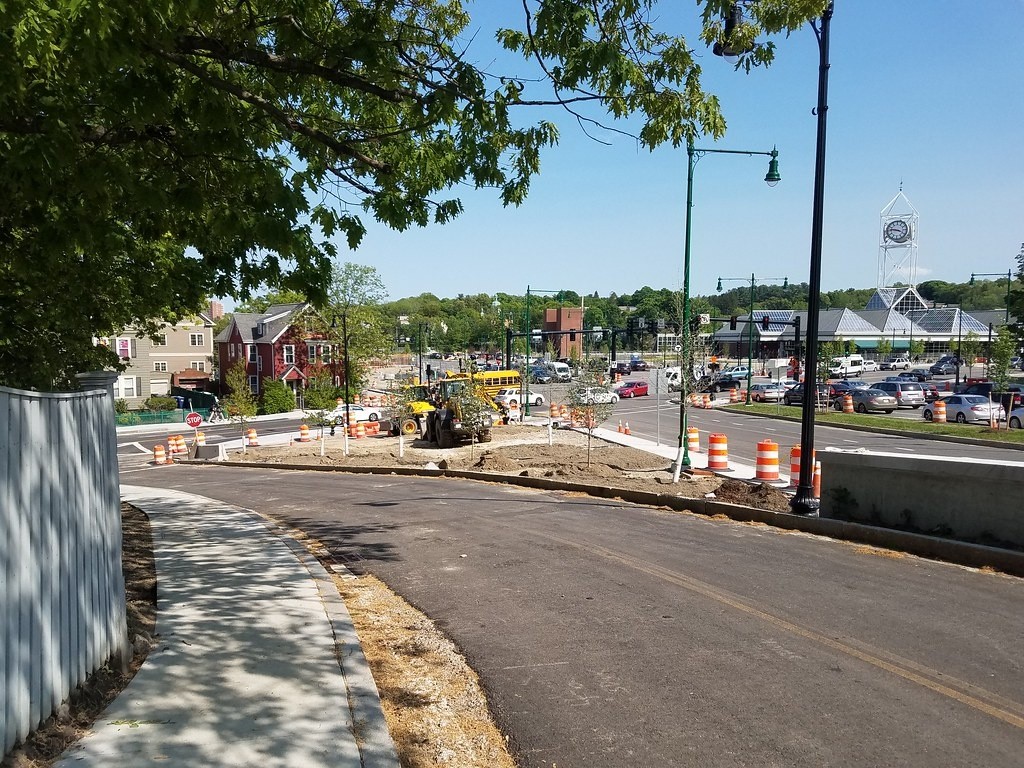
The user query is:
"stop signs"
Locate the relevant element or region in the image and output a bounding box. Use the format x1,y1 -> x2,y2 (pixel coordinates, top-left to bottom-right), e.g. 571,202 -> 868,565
185,412 -> 202,427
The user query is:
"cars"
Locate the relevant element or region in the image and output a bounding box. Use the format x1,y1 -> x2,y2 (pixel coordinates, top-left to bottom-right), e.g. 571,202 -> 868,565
316,403 -> 382,426
578,387 -> 620,406
613,381 -> 649,399
555,356 -> 583,370
430,352 -> 552,384
666,356 -> 1024,429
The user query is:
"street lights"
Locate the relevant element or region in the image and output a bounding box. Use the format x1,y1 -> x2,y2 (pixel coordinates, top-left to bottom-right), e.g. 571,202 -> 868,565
892,328 -> 906,352
418,322 -> 429,393
525,284 -> 564,416
969,269 -> 1011,323
330,310 -> 351,426
716,272 -> 788,406
501,311 -> 527,370
674,128 -> 781,465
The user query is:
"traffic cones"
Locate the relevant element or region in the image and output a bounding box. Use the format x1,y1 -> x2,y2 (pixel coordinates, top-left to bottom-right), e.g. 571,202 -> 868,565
316,430 -> 322,441
571,415 -> 578,428
387,428 -> 392,437
623,421 -> 633,435
617,420 -> 623,433
991,413 -> 997,429
289,434 -> 294,447
166,443 -> 174,464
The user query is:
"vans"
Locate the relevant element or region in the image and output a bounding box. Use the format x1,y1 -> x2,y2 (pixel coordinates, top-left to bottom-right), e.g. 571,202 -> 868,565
548,361 -> 571,383
590,356 -> 609,372
826,356 -> 865,378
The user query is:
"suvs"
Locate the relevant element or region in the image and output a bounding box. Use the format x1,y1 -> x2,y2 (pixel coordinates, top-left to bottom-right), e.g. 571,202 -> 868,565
494,387 -> 545,407
630,360 -> 646,372
879,358 -> 910,372
611,363 -> 631,375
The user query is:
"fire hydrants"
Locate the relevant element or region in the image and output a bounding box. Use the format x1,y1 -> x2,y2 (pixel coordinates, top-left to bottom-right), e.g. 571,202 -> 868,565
330,424 -> 336,436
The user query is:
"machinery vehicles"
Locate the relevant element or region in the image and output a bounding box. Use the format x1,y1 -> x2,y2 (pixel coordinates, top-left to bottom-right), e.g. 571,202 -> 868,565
389,370 -> 509,449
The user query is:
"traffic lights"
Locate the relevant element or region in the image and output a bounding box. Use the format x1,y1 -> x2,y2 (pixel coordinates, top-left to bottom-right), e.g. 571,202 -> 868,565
729,315 -> 737,330
763,316 -> 769,331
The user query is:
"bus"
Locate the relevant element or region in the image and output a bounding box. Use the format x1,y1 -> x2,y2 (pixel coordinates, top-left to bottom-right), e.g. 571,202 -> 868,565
472,369 -> 523,400
